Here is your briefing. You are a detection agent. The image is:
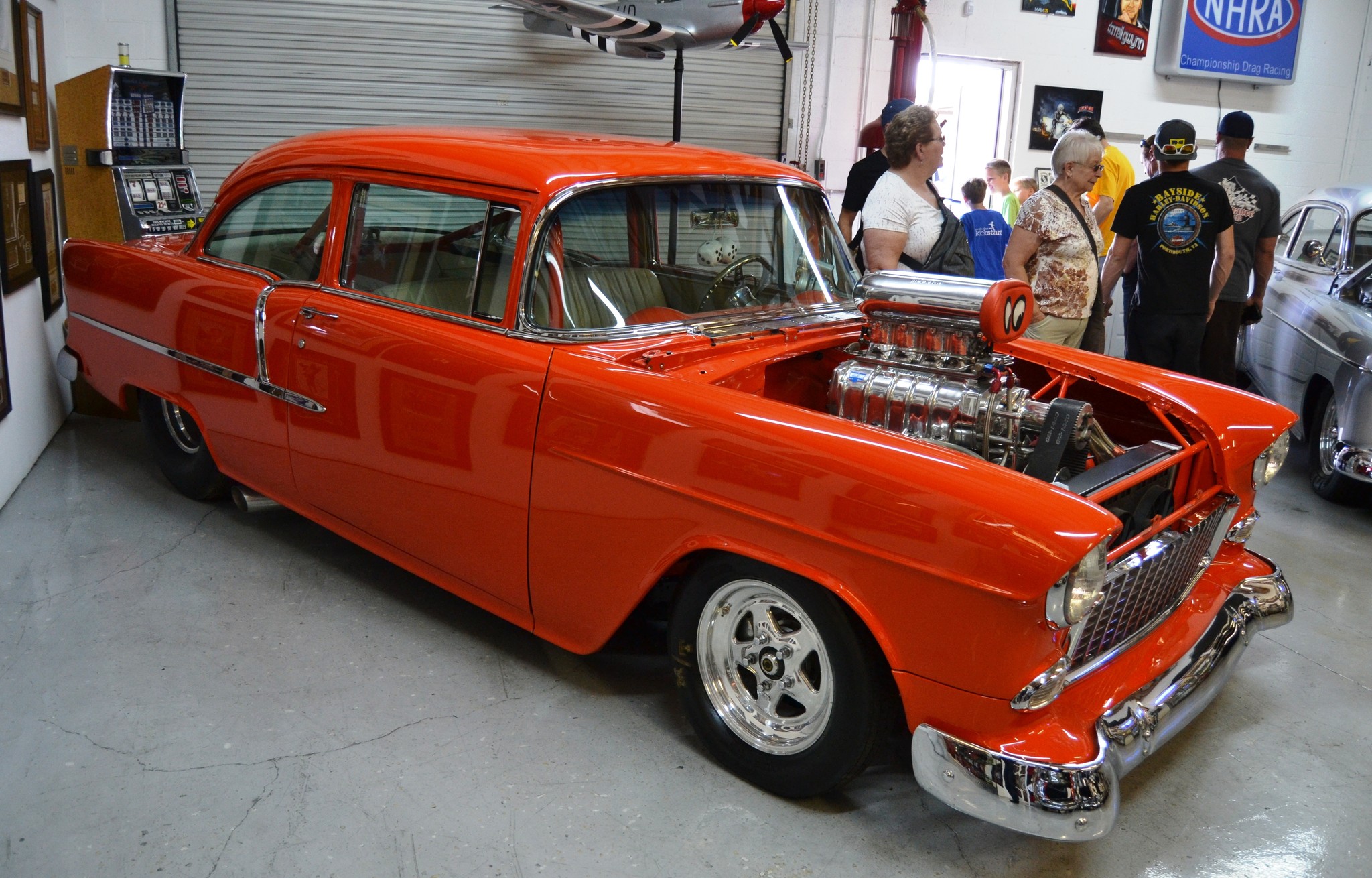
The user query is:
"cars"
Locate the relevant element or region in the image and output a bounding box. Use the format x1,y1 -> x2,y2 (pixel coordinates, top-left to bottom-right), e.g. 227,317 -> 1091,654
55,124 -> 1297,845
1236,184 -> 1372,508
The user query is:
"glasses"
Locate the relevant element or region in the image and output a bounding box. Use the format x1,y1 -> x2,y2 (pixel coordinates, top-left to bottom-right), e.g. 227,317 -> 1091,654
928,135 -> 946,145
1140,139 -> 1151,151
1154,139 -> 1196,155
1062,159 -> 1104,172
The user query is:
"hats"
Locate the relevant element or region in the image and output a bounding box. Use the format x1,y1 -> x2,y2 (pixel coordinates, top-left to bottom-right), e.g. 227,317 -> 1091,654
881,98 -> 915,127
1216,109 -> 1254,139
1154,119 -> 1197,161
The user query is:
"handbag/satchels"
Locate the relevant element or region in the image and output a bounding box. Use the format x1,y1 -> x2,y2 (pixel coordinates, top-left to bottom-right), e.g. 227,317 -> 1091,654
921,201 -> 975,280
1079,280 -> 1105,355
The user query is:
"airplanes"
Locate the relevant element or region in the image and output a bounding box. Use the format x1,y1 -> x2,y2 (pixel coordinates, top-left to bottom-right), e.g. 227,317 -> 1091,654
489,0 -> 809,61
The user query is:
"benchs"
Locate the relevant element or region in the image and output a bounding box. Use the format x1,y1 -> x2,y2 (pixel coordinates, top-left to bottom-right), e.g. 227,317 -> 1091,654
373,262 -> 667,332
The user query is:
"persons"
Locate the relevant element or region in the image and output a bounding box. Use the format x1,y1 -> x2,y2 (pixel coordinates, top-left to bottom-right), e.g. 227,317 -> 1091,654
837,99 -> 915,258
860,104 -> 946,272
1002,129 -> 1104,349
1009,176 -> 1039,207
960,177 -> 1012,281
1071,110 -> 1283,389
986,158 -> 1020,230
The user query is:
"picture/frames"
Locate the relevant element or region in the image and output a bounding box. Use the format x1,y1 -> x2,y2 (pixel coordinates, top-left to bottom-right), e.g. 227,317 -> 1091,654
26,168 -> 64,322
0,159 -> 39,295
20,0 -> 50,151
